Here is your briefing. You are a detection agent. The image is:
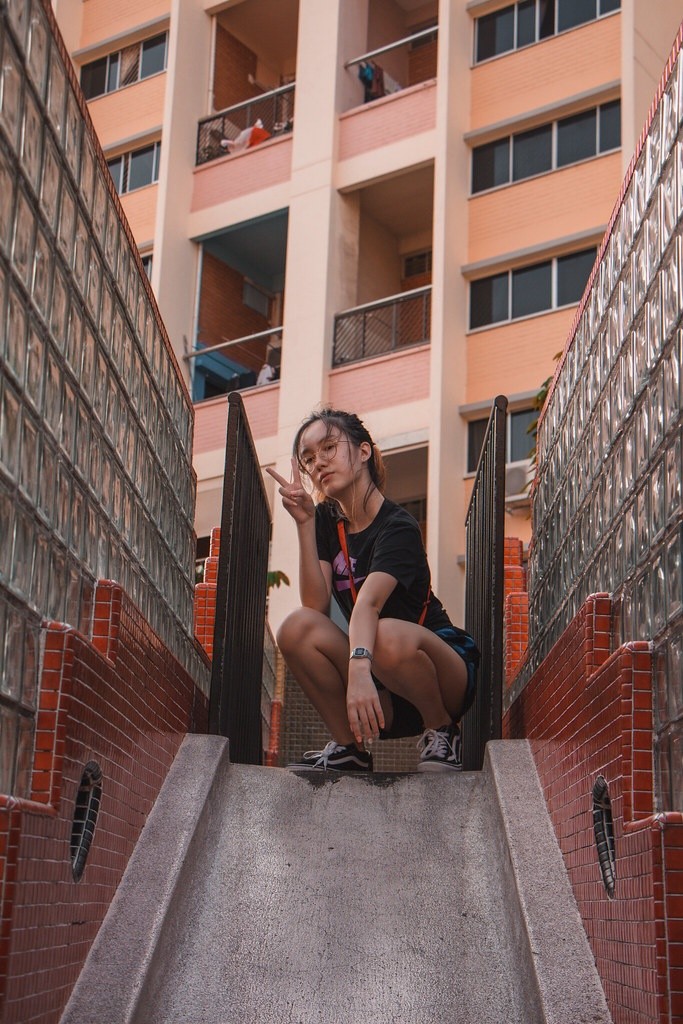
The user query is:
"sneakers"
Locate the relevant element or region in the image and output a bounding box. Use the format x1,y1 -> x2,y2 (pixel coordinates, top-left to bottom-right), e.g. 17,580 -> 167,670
416,721 -> 462,773
288,740 -> 374,774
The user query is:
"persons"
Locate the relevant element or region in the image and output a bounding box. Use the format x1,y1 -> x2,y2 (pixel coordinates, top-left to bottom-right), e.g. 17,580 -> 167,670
264,406 -> 485,775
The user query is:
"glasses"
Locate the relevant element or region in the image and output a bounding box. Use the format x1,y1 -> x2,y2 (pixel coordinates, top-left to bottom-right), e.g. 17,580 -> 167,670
298,440 -> 355,475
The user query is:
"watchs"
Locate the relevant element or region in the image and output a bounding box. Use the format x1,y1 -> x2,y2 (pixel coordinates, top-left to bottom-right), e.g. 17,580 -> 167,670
348,647 -> 375,665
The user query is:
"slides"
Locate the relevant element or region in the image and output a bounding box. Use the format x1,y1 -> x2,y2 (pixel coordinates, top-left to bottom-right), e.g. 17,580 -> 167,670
59,736 -> 620,1024
